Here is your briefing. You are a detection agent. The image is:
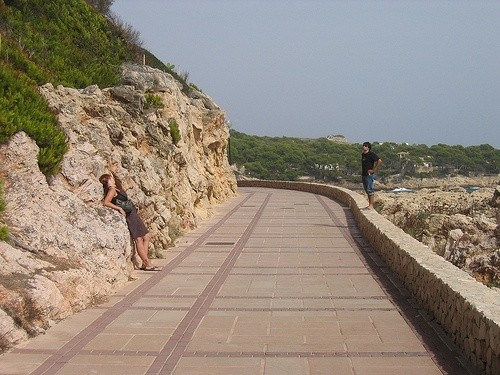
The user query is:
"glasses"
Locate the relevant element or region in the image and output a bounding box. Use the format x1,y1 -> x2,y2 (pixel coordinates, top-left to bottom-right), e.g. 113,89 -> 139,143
363,145 -> 368,147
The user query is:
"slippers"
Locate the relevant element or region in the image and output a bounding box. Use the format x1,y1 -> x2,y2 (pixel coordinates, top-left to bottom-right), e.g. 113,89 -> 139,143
141,263 -> 164,271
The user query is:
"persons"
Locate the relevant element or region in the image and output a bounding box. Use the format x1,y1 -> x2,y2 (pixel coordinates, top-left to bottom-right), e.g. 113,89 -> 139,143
361,142 -> 382,209
99,174 -> 162,271
